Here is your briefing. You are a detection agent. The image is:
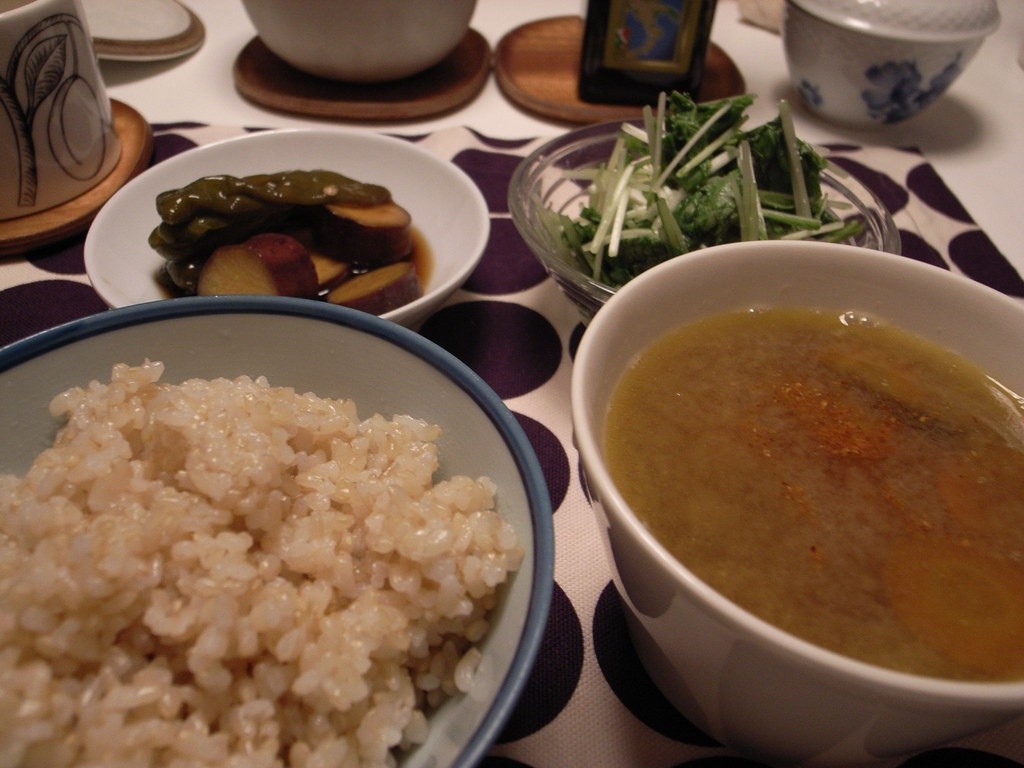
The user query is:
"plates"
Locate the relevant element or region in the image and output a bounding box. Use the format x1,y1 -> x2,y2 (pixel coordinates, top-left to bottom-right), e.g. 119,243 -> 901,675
79,0 -> 205,62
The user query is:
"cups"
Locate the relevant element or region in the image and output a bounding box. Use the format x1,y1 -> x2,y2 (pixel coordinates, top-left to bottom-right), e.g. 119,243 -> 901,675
0,0 -> 122,218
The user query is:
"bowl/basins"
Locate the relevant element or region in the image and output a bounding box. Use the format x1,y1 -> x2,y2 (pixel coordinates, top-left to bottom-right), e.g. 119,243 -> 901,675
85,127 -> 491,332
0,294 -> 555,768
508,120 -> 902,328
570,240 -> 1024,768
240,0 -> 477,77
781,0 -> 1001,131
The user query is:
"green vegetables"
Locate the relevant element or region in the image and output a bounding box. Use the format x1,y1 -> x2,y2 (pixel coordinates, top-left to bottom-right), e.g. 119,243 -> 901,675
525,89 -> 861,290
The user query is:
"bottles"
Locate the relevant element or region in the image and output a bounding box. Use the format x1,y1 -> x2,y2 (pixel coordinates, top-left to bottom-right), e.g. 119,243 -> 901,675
578,0 -> 718,110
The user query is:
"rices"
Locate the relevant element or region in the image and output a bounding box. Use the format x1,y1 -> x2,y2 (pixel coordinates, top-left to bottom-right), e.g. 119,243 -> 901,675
0,359 -> 526,768
